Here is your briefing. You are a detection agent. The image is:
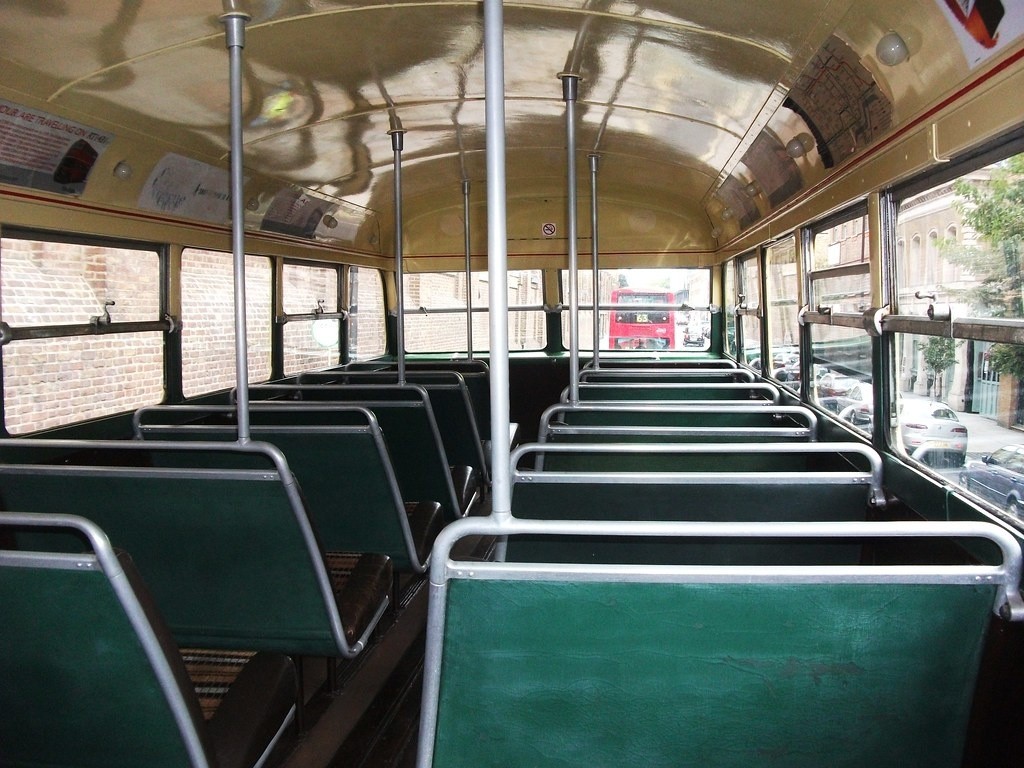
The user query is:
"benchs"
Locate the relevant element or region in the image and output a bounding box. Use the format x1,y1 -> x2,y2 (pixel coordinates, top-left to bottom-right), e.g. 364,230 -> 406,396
0,357 -> 1024,768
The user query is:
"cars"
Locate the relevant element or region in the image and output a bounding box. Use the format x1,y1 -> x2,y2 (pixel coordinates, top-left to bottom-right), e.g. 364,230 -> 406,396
960,446 -> 1023,516
868,398 -> 968,465
835,384 -> 905,424
750,353 -> 830,390
676,317 -> 755,353
815,373 -> 862,409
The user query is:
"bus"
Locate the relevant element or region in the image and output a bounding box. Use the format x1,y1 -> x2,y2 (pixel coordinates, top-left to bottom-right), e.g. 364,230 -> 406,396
608,288 -> 675,349
